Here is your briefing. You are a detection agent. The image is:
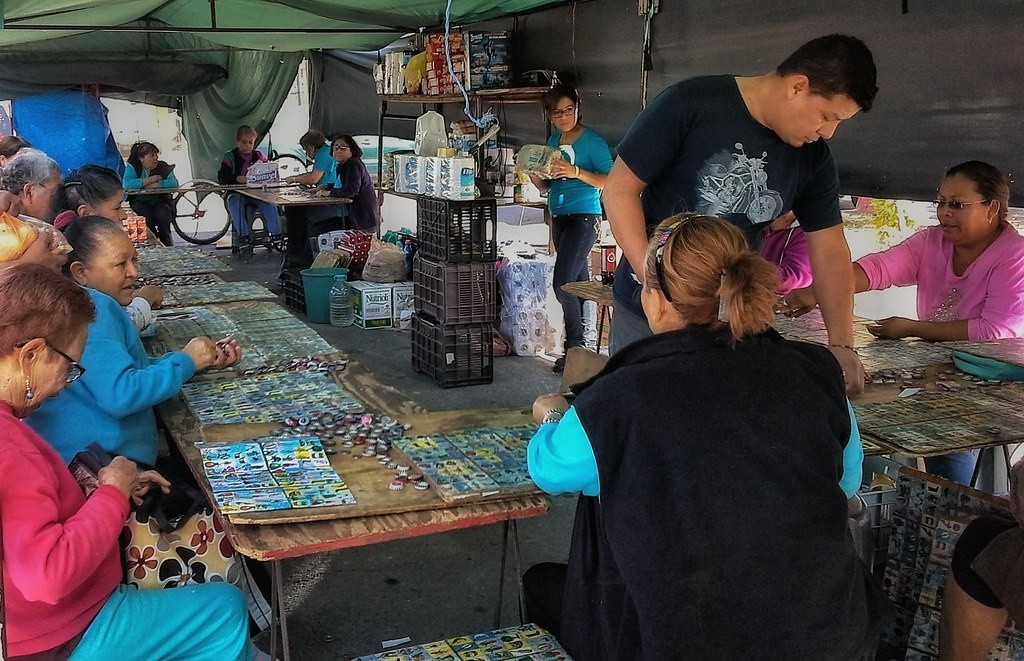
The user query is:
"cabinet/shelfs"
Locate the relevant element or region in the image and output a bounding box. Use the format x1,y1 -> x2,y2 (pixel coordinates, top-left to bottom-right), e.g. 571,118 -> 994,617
374,87 -> 553,252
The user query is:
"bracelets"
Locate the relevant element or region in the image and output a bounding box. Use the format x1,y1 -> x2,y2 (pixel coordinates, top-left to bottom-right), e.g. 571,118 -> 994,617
543,409 -> 565,424
829,343 -> 859,355
293,177 -> 296,183
575,166 -> 580,177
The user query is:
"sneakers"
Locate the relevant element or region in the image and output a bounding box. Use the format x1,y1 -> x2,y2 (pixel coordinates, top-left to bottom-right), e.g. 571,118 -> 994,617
270,235 -> 284,243
239,237 -> 251,249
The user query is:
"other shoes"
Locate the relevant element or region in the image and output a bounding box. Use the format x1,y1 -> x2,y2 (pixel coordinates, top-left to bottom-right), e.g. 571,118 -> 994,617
553,364 -> 565,374
555,356 -> 565,364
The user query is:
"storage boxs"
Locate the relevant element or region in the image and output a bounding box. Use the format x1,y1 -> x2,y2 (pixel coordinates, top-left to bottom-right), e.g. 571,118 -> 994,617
852,456 -> 904,579
283,121 -> 617,387
374,28 -> 512,95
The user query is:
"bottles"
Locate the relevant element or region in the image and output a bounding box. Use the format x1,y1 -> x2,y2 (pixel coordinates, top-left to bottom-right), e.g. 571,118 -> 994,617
381,225 -> 412,269
487,162 -> 499,184
328,275 -> 354,326
514,165 -> 531,203
412,108 -> 447,156
600,229 -> 616,285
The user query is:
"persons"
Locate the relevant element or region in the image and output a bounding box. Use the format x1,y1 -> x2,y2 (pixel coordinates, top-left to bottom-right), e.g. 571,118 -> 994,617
1,134 -> 163,332
120,142 -> 179,248
937,515 -> 1024,661
771,162 -> 1023,492
521,211 -> 893,660
512,85 -> 613,373
0,259 -> 253,661
284,129 -> 380,252
757,209 -> 813,297
603,34 -> 877,394
217,125 -> 283,248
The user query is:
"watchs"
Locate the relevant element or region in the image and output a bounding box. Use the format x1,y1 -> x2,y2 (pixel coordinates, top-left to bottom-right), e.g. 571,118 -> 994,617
24,213 -> 241,469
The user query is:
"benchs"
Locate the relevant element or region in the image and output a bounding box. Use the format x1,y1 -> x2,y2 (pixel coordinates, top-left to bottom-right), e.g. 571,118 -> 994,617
352,623 -> 575,661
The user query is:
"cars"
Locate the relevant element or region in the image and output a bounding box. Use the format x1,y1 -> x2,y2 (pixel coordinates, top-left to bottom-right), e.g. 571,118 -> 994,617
256,136 -> 418,188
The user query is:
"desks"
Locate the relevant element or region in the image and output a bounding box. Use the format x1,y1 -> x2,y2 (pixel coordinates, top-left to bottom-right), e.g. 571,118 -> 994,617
558,277 -> 1024,492
233,189 -> 353,292
122,230 -> 575,661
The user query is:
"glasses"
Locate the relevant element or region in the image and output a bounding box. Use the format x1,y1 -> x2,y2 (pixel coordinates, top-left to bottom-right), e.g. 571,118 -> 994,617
551,105 -> 575,119
333,145 -> 350,151
932,200 -> 989,208
16,337 -> 86,383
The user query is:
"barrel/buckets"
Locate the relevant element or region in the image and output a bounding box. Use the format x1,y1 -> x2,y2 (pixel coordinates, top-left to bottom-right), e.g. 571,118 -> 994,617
300,268 -> 349,323
